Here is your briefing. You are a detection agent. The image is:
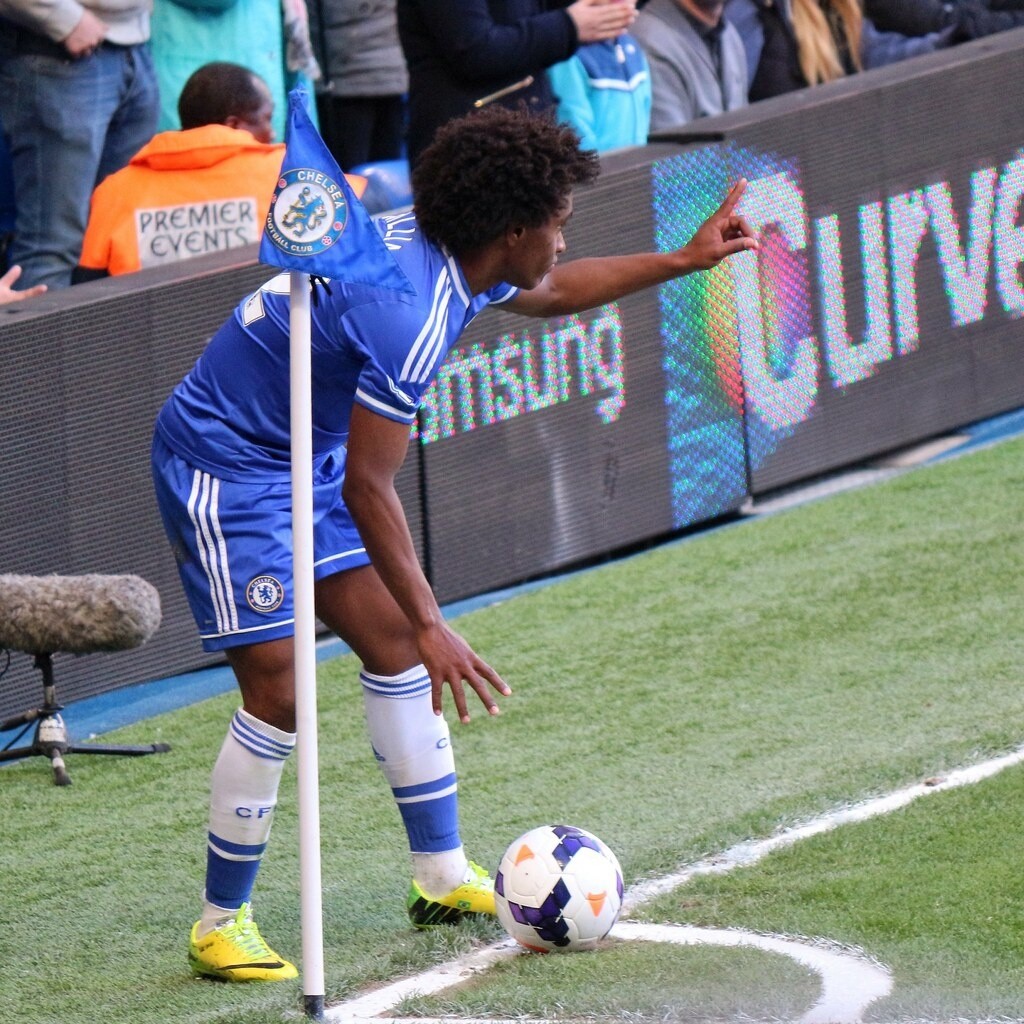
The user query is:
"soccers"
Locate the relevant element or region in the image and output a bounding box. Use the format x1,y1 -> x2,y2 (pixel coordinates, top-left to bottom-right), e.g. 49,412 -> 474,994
494,823 -> 625,953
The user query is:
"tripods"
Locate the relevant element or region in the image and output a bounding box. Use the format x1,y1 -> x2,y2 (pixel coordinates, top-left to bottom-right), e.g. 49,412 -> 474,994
0,653 -> 171,788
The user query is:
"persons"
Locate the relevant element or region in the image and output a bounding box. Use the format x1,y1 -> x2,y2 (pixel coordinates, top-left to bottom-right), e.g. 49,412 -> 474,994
0,0 -> 1024,305
151,106 -> 759,982
72,60 -> 367,285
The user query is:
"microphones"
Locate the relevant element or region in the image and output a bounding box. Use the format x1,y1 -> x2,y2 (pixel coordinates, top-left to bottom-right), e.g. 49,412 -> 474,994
0,573 -> 162,654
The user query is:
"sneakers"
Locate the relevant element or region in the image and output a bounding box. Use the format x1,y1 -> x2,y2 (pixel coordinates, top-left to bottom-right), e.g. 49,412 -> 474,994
408,861 -> 499,930
187,903 -> 300,982
